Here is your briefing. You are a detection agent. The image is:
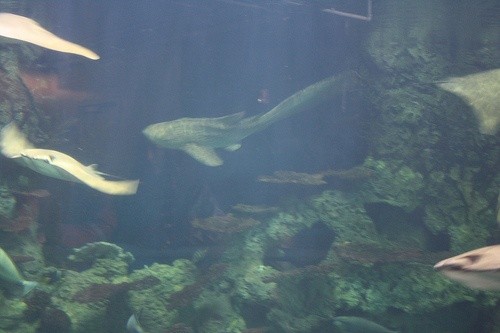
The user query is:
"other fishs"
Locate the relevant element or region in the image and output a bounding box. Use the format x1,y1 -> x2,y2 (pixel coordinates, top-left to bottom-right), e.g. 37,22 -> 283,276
1,1 -> 498,332
